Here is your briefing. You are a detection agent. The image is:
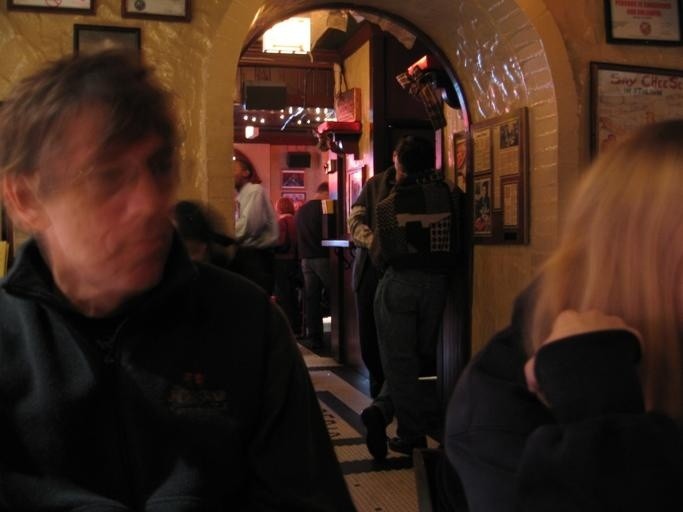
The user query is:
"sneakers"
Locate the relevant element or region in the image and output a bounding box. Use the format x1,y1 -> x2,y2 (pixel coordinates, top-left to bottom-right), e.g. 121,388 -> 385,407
361,404 -> 388,461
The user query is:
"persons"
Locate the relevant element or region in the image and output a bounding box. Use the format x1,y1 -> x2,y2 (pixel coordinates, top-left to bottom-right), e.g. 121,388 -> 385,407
443,118 -> 683,508
229,148 -> 280,289
360,134 -> 468,466
475,179 -> 490,220
348,144 -> 405,401
276,198 -> 297,258
0,43 -> 356,508
294,182 -> 331,355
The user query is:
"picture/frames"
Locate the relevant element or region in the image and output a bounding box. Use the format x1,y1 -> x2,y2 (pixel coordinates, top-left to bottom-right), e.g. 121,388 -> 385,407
281,171 -> 305,188
280,192 -> 307,201
120,1 -> 191,23
452,107 -> 535,246
72,24 -> 143,67
605,0 -> 683,44
345,164 -> 368,234
8,1 -> 93,14
589,64 -> 682,163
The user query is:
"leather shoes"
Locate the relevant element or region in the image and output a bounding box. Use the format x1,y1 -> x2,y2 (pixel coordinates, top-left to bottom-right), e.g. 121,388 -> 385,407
389,435 -> 428,455
